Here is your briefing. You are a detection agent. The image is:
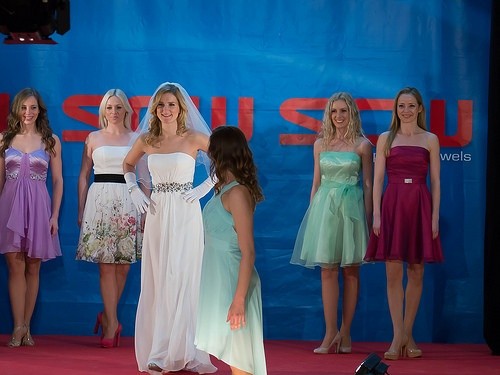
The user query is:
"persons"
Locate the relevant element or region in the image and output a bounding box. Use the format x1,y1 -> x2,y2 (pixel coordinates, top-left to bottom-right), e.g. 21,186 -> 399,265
364,87 -> 443,361
194,126 -> 267,375
290,93 -> 373,353
78,89 -> 151,348
0,88 -> 63,347
122,82 -> 220,375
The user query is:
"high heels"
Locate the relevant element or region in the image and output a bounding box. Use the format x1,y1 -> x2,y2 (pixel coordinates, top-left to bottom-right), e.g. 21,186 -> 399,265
94,313 -> 106,344
313,330 -> 342,354
384,336 -> 408,359
406,337 -> 421,357
23,325 -> 35,346
102,322 -> 122,348
340,334 -> 352,352
8,324 -> 28,349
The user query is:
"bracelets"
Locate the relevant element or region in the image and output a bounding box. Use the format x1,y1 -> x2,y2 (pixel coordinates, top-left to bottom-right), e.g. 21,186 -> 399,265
128,185 -> 139,193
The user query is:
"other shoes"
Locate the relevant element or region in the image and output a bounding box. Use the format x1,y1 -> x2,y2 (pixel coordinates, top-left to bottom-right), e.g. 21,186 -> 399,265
148,363 -> 162,372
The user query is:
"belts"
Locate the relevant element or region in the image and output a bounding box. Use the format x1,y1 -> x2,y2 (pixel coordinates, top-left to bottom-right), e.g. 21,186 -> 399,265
93,174 -> 126,184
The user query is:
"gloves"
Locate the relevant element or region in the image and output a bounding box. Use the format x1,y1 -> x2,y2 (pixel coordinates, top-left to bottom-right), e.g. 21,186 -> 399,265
124,172 -> 150,215
182,173 -> 218,204
